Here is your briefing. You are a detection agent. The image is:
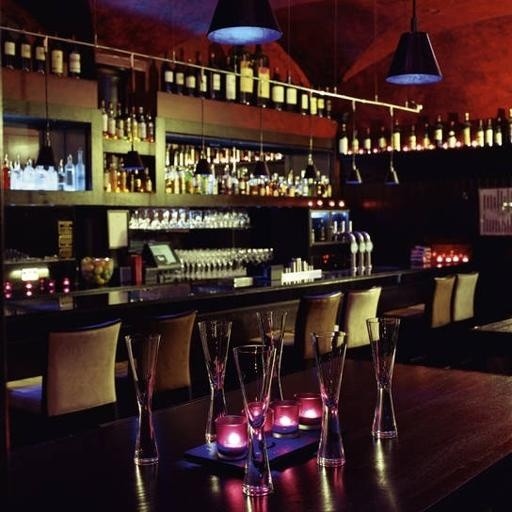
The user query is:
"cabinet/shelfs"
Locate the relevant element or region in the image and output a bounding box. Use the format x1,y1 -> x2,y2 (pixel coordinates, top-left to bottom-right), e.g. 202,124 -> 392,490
0,25 -> 338,286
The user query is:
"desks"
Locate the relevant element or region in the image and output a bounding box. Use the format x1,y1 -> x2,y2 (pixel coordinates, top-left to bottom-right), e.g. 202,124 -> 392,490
1,356 -> 511,512
474,318 -> 512,340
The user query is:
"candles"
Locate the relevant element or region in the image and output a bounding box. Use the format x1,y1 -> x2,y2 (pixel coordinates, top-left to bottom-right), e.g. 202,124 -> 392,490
297,394 -> 323,424
215,414 -> 249,453
241,404 -> 274,435
272,400 -> 299,435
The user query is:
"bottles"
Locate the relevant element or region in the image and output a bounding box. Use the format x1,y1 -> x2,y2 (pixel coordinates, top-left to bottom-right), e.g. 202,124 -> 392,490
98,97 -> 156,143
103,151 -> 153,193
1,146 -> 88,192
0,23 -> 82,80
232,318 -> 400,496
312,217 -> 354,244
124,309 -> 291,468
160,44 -> 335,119
337,111 -> 510,156
169,147 -> 333,199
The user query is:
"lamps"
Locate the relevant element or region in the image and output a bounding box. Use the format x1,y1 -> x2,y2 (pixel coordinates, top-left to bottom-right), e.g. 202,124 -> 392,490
206,0 -> 283,46
386,0 -> 442,85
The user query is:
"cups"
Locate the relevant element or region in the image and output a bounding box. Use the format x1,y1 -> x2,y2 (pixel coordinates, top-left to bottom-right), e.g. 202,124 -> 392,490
215,393 -> 323,462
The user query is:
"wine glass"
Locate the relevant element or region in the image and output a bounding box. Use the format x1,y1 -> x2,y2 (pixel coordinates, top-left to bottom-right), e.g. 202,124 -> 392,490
244,248 -> 276,269
190,209 -> 252,229
174,248 -> 247,279
128,208 -> 190,230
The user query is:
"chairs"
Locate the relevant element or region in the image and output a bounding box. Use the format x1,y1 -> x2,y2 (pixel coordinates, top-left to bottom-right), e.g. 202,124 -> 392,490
4,317 -> 124,453
385,276 -> 455,329
249,292 -> 345,361
115,310 -> 198,408
335,285 -> 383,350
408,272 -> 479,323
